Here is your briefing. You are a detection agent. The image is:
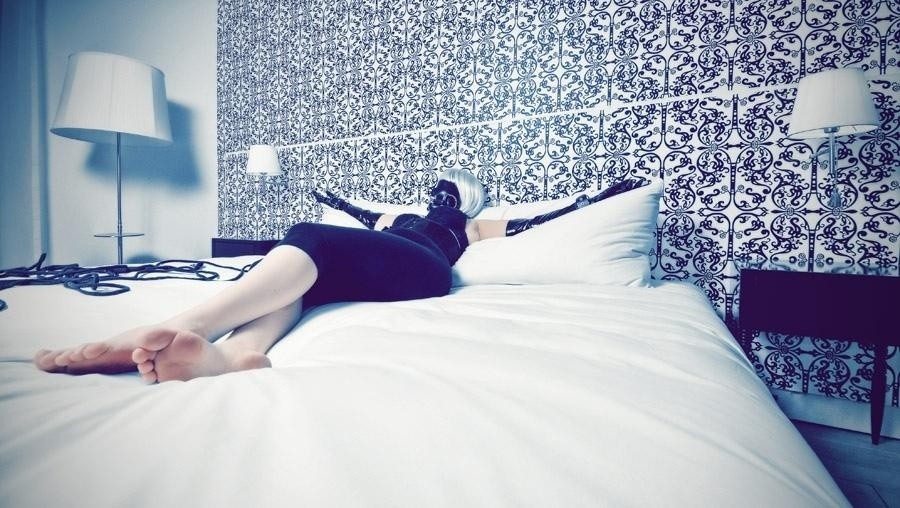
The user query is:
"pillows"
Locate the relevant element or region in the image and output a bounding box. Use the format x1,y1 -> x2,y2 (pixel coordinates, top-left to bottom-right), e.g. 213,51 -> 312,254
318,177 -> 665,289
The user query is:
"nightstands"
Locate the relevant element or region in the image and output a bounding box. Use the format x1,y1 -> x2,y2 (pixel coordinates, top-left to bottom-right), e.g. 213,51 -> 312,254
737,266 -> 900,445
209,236 -> 282,258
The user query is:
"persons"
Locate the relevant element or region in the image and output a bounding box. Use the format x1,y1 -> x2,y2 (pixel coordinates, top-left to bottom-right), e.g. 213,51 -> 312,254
32,166 -> 651,386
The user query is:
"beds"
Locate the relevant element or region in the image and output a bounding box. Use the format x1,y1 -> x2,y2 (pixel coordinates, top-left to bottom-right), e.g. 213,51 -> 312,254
0,254 -> 853,507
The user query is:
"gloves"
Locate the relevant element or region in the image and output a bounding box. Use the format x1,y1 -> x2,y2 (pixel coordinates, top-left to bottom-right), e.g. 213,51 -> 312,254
506,178 -> 643,235
313,191 -> 384,230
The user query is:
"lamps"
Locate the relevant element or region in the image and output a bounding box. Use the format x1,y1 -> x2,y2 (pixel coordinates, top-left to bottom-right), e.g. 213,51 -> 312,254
244,141 -> 283,237
46,49 -> 173,259
782,64 -> 882,271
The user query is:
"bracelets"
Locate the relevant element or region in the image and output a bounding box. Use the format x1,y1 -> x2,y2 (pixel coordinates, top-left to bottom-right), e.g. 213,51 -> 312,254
575,193 -> 592,209
